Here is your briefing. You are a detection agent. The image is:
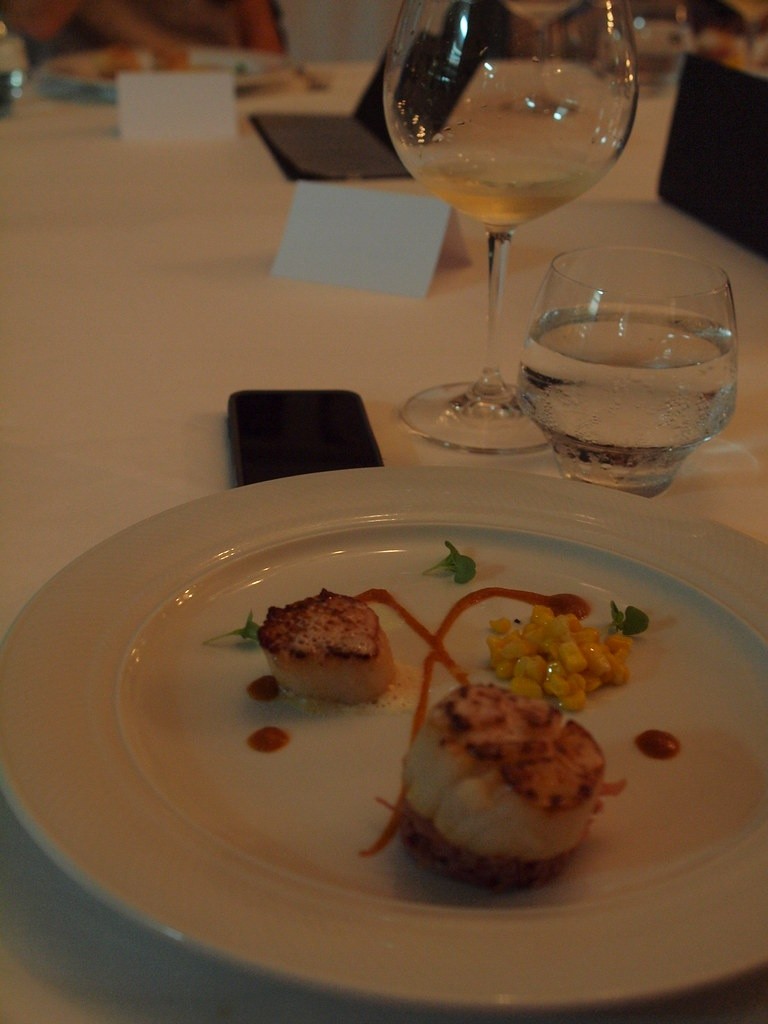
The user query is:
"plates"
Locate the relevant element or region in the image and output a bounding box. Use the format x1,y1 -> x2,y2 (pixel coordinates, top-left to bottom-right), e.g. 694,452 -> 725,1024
0,459 -> 768,1020
40,41 -> 297,95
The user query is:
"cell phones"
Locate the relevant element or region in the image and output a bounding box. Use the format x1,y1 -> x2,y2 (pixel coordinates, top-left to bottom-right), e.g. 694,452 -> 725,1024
228,389 -> 383,485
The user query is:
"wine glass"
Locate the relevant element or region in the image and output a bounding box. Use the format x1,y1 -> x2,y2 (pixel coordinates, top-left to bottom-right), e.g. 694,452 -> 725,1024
381,0 -> 639,457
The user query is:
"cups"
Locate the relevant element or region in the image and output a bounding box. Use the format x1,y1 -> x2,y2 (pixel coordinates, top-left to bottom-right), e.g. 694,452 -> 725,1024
518,248 -> 739,498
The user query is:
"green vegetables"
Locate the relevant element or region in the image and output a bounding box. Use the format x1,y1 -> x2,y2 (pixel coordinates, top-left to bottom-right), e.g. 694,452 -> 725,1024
204,608 -> 261,642
423,541 -> 476,583
609,600 -> 649,635
